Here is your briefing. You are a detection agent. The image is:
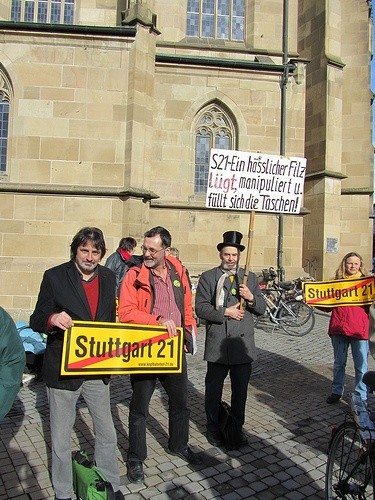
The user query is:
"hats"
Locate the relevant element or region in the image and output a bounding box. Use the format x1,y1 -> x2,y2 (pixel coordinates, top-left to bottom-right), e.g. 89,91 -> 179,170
217,231 -> 245,252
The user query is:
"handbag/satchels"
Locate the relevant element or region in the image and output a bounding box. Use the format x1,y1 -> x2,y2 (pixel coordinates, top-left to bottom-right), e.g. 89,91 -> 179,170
72,449 -> 116,500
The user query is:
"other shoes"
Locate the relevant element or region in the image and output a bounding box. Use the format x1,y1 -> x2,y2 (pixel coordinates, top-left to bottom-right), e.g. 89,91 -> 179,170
325,393 -> 341,405
226,430 -> 241,450
205,427 -> 225,447
114,490 -> 126,500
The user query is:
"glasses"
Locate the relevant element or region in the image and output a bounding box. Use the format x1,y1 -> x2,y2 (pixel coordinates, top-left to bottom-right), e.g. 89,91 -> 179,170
141,245 -> 170,254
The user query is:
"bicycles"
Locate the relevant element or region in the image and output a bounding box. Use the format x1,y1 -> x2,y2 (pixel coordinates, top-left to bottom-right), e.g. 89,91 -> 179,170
325,412 -> 375,500
254,267 -> 315,337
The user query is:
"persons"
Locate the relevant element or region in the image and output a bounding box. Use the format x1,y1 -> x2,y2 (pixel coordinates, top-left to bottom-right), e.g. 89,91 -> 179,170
0,306 -> 27,421
168,248 -> 192,290
193,229 -> 268,451
29,226 -> 126,500
103,236 -> 137,322
117,226 -> 198,485
301,251 -> 375,406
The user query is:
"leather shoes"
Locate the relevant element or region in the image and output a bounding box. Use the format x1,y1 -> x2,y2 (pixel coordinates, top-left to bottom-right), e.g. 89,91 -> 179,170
126,458 -> 145,484
164,442 -> 195,463
